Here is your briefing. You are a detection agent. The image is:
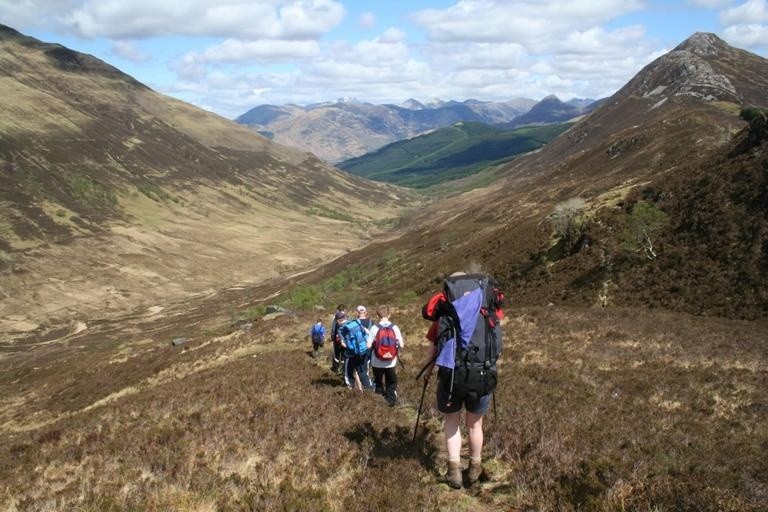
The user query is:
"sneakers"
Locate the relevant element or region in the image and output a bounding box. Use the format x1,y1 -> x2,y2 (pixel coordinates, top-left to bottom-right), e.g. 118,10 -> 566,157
445,459 -> 462,490
468,458 -> 485,488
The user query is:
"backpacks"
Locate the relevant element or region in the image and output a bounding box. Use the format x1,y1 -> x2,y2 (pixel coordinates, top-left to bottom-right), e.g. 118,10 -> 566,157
421,272 -> 503,395
372,323 -> 399,363
342,320 -> 370,356
311,325 -> 323,347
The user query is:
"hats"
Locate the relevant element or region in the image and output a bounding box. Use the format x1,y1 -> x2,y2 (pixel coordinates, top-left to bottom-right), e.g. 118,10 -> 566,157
333,311 -> 348,321
356,305 -> 366,313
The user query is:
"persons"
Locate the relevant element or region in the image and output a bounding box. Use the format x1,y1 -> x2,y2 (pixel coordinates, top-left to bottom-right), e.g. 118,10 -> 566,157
365,304 -> 405,406
312,319 -> 325,357
423,271 -> 489,490
352,306 -> 375,393
334,312 -> 374,390
330,303 -> 349,373
